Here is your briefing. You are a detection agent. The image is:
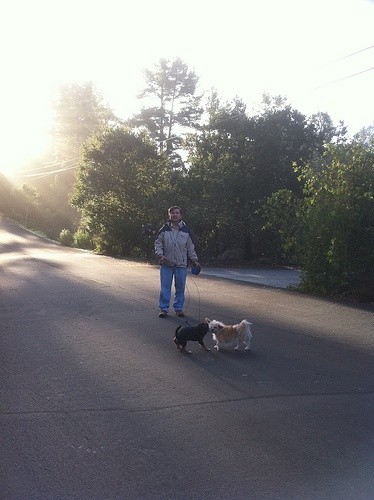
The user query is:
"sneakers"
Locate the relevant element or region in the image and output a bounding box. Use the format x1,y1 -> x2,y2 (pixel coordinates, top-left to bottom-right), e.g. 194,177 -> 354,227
159,310 -> 167,317
176,310 -> 184,317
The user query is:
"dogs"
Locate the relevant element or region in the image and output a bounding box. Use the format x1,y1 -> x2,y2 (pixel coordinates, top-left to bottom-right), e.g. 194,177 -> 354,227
172,322 -> 211,354
206,318 -> 254,352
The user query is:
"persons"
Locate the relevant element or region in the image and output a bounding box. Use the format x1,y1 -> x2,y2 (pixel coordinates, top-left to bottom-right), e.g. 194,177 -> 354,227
154,206 -> 200,317
